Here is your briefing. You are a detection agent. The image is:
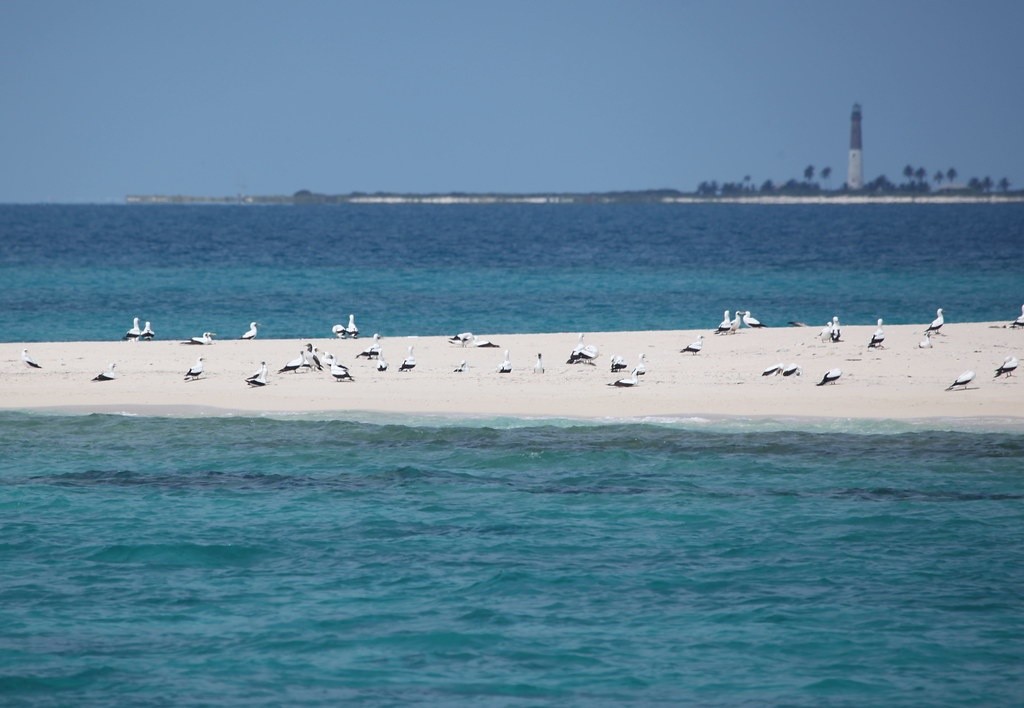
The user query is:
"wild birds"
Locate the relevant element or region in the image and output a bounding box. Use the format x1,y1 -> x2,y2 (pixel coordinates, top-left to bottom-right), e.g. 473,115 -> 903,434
345,314 -> 360,339
21,348 -> 42,369
476,339 -> 500,348
246,361 -> 269,387
184,332 -> 216,345
322,351 -> 355,383
90,362 -> 117,381
398,345 -> 417,372
332,324 -> 346,338
355,333 -> 382,360
240,321 -> 261,340
301,343 -> 324,373
497,302 -> 1024,391
126,317 -> 143,341
449,332 -> 474,348
184,358 -> 207,381
277,350 -> 305,374
139,321 -> 155,340
453,360 -> 470,373
376,351 -> 388,371
